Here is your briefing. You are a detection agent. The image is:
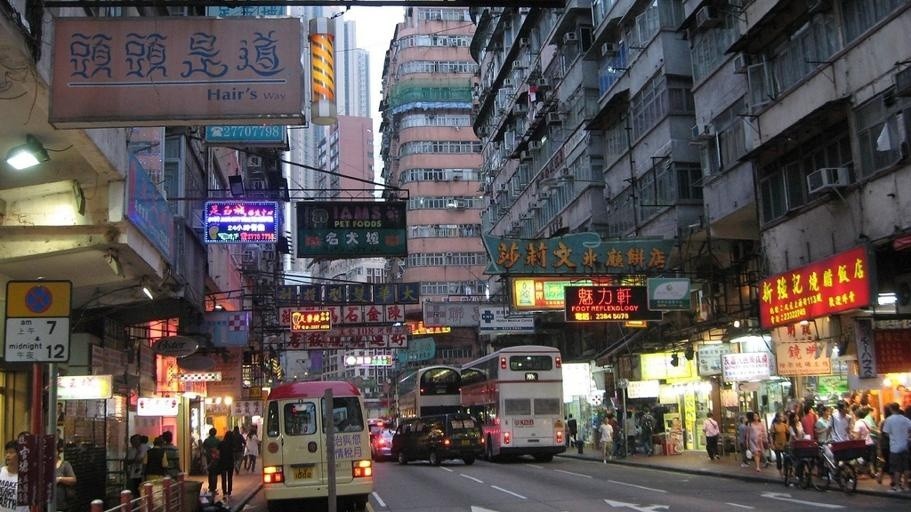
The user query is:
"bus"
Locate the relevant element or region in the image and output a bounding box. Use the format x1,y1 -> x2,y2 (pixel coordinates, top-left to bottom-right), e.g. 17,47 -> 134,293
366,419 -> 383,447
461,344 -> 567,464
390,367 -> 460,422
260,378 -> 371,510
462,368 -> 489,421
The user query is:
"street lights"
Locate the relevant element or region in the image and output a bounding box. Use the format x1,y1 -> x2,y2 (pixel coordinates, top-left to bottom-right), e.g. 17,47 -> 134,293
615,377 -> 632,461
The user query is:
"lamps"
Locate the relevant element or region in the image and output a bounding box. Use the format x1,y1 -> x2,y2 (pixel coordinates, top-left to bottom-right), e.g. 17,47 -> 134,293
69,180 -> 162,303
671,339 -> 695,367
229,174 -> 245,196
2,134 -> 50,172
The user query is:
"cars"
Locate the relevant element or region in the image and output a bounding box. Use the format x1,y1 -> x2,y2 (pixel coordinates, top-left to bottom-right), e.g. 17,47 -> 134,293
371,430 -> 401,462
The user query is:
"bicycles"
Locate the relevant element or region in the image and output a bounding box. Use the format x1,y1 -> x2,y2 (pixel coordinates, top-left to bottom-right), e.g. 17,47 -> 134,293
808,439 -> 857,495
776,440 -> 810,490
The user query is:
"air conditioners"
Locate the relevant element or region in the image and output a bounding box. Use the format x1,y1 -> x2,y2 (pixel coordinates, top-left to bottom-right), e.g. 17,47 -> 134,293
731,55 -> 745,72
691,123 -> 717,142
442,7 -> 578,258
600,43 -> 615,58
696,7 -> 721,27
249,155 -> 279,192
806,167 -> 849,195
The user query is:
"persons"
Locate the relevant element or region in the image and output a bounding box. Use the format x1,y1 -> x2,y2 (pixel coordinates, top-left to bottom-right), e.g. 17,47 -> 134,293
568,413 -> 577,444
217,431 -> 236,495
564,416 -> 569,446
126,431 -> 180,492
702,413 -> 720,461
233,426 -> 259,474
58,403 -> 65,421
740,385 -> 911,493
598,417 -> 614,463
0,440 -> 31,512
57,429 -> 63,449
56,449 -> 78,511
18,432 -> 32,444
640,408 -> 656,455
202,428 -> 220,491
626,411 -> 638,455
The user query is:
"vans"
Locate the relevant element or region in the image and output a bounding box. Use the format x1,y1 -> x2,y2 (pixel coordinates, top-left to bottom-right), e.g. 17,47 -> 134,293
387,414 -> 479,469
